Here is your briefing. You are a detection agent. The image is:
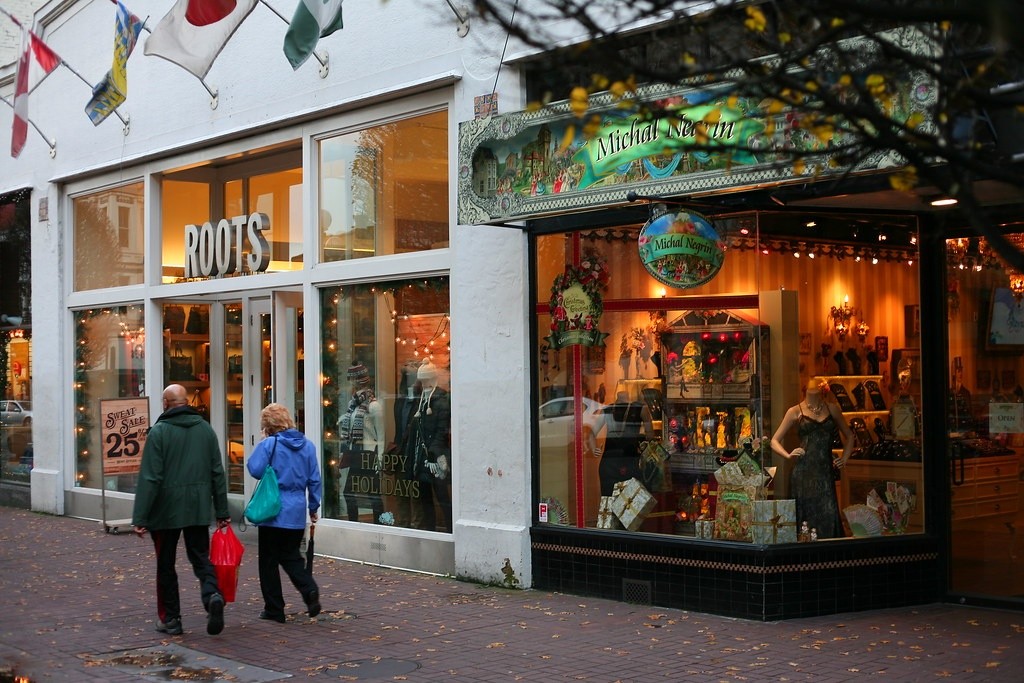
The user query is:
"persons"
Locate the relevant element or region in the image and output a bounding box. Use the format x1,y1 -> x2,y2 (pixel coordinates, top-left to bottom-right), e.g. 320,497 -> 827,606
393,357 -> 452,533
337,360 -> 385,526
770,378 -> 855,540
688,414 -> 744,450
246,402 -> 322,622
667,353 -> 689,399
587,391 -> 656,496
130,384 -> 231,636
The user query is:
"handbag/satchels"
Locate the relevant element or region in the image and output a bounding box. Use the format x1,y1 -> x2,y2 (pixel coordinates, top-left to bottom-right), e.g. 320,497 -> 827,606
209,523 -> 244,603
238,437 -> 280,533
429,461 -> 451,481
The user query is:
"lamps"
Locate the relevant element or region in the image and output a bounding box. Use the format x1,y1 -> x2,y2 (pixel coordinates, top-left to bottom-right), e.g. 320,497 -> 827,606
826,293 -> 869,347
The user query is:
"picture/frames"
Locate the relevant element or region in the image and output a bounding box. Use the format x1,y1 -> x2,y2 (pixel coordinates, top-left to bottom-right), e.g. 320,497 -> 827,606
875,336 -> 888,362
587,344 -> 606,373
798,333 -> 809,355
985,280 -> 1024,355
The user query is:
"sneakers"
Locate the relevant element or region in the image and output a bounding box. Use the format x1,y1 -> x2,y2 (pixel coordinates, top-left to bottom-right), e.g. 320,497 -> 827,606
206,593 -> 225,636
156,617 -> 182,634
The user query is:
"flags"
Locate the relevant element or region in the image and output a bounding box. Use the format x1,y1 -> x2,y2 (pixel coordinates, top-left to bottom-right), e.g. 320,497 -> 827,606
11,24 -> 63,159
143,0 -> 260,80
283,0 -> 343,71
84,1 -> 144,126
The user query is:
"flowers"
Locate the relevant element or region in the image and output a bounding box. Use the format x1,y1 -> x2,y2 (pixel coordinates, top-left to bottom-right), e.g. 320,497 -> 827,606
619,326 -> 650,359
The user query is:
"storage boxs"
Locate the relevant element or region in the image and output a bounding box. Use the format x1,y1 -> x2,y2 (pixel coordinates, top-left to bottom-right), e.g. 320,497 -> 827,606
696,483 -> 799,544
596,476 -> 658,532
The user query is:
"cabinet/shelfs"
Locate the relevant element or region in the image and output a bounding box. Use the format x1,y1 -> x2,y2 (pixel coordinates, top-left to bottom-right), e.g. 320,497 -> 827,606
121,330 -> 303,468
839,451 -> 1021,529
814,375 -> 891,459
892,348 -> 921,415
659,308 -> 771,476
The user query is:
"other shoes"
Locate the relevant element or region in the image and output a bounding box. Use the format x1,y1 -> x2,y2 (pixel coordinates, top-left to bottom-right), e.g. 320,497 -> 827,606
304,589 -> 321,617
259,605 -> 285,622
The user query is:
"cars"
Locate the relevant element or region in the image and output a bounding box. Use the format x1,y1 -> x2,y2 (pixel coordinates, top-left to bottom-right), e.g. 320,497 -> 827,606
538,396 -> 616,457
0,400 -> 32,427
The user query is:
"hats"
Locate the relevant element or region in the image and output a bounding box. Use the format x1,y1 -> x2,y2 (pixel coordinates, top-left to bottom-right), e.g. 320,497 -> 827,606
414,357 -> 439,418
346,361 -> 370,385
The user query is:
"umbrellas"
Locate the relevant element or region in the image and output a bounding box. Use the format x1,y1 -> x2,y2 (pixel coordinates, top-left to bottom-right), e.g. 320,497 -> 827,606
306,525 -> 315,576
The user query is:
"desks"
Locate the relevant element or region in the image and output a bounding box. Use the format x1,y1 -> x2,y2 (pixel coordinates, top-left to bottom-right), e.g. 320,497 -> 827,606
613,379 -> 661,400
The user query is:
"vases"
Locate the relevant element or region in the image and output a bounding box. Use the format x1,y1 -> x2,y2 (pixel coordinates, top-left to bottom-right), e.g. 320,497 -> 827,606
635,347 -> 644,380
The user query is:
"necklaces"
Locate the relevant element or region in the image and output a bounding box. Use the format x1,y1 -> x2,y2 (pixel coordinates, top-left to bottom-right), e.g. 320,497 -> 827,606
804,399 -> 824,416
612,401 -> 630,438
